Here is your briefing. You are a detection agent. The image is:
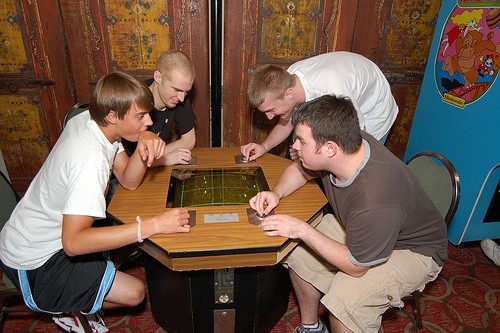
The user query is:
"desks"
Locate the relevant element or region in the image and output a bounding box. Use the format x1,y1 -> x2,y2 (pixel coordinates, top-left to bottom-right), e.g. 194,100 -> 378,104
105,147 -> 328,333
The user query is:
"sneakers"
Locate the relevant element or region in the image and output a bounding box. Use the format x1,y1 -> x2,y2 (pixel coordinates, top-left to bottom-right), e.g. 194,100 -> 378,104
292,317 -> 329,333
52,309 -> 109,333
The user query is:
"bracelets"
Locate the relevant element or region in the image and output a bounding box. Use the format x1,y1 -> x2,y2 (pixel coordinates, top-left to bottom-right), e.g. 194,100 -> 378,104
135,215 -> 144,243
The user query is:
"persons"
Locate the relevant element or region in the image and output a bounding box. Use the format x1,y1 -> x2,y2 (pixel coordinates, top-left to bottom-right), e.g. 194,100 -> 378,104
91,51 -> 197,269
248,94 -> 449,331
481,238 -> 500,267
0,71 -> 190,332
239,51 -> 398,214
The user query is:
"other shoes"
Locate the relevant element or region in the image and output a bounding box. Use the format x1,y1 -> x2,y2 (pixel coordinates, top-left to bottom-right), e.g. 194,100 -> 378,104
480,239 -> 500,267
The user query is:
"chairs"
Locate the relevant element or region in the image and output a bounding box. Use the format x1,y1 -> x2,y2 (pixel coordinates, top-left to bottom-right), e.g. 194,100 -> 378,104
0,170 -> 94,333
402,152 -> 460,330
